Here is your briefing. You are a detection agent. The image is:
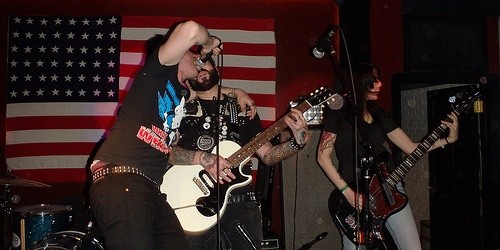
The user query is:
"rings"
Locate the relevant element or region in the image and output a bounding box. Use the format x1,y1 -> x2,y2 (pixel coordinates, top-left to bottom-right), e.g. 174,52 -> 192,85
250,104 -> 254,107
222,165 -> 226,171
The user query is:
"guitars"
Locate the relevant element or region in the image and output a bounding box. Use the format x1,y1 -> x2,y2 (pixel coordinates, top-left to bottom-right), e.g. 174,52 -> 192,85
160,86 -> 339,235
327,86 -> 481,247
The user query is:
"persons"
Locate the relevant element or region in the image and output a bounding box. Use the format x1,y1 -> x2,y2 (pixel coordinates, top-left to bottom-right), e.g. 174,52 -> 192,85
316,61 -> 459,250
168,46 -> 309,250
89,20 -> 256,250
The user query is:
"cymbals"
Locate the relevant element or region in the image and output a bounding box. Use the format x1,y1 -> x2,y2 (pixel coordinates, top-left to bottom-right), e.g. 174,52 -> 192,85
0,173 -> 52,187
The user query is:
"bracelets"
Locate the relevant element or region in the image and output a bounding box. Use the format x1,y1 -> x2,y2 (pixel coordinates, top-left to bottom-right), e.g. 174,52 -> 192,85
230,88 -> 236,96
290,136 -> 306,151
340,185 -> 349,194
438,139 -> 444,148
445,137 -> 450,144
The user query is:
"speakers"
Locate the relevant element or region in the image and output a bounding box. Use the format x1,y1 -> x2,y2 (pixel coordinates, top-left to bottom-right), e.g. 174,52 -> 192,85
277,127 -> 345,250
188,190 -> 265,250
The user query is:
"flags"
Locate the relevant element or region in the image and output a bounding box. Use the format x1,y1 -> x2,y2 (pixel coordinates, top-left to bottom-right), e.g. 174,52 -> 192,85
5,15 -> 277,180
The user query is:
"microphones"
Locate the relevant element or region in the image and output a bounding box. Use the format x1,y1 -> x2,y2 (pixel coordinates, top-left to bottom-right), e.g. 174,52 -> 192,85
195,42 -> 222,66
0,194 -> 21,203
312,26 -> 340,59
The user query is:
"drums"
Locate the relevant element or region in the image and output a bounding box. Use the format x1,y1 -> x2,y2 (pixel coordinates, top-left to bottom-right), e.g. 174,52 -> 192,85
34,231 -> 105,250
10,205 -> 73,250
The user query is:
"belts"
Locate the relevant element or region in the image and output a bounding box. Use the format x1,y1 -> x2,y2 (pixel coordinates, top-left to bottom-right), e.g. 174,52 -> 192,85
92,166 -> 160,190
205,192 -> 256,208
